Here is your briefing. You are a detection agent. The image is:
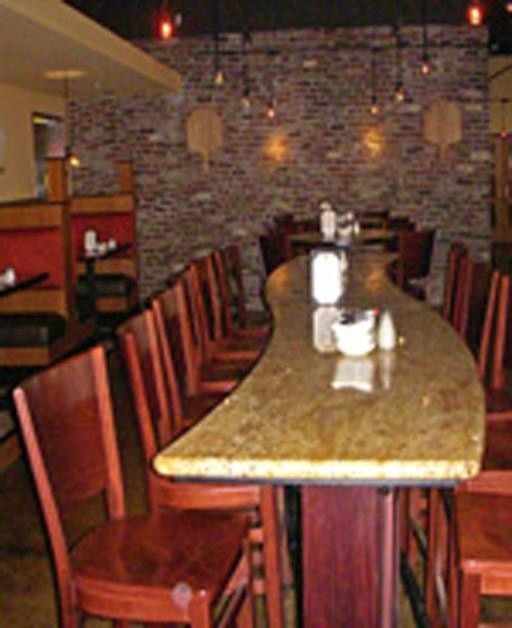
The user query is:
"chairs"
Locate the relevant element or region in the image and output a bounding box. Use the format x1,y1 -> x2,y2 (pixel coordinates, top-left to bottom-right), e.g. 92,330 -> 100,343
152,280 -> 238,437
455,258 -> 474,339
397,229 -> 422,292
474,269 -> 512,390
119,311 -> 291,627
188,254 -> 266,379
403,414 -> 511,610
219,247 -> 274,340
433,242 -> 455,318
260,195 -> 413,248
18,346 -> 256,628
442,488 -> 512,628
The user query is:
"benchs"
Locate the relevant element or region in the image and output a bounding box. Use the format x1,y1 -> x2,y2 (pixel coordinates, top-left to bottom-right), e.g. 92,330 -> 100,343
67,193 -> 142,330
3,199 -> 75,389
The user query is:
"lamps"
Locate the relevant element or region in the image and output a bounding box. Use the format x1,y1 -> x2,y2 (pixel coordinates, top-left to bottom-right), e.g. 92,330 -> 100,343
392,32 -> 406,100
214,41 -> 224,85
239,46 -> 252,107
184,97 -> 227,173
419,25 -> 431,75
419,96 -> 512,162
266,56 -> 276,118
369,48 -> 380,113
